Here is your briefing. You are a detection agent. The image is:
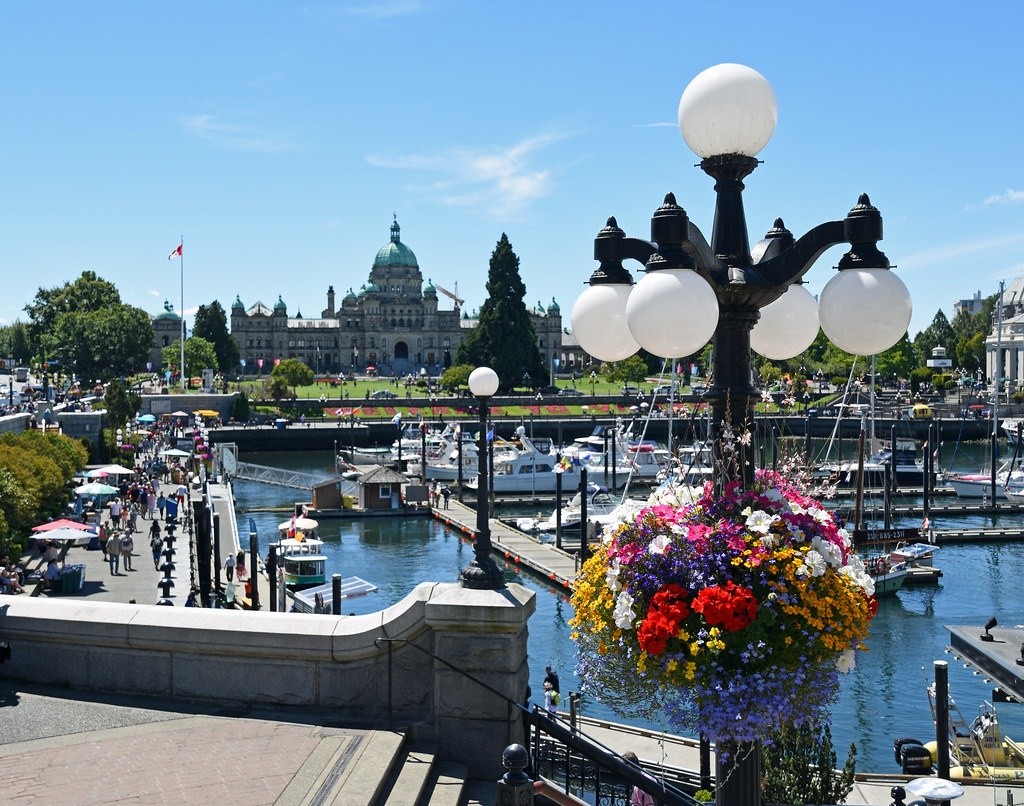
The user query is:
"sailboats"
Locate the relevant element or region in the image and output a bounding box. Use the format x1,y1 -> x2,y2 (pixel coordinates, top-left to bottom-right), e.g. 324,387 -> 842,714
832,420 -> 909,598
944,278 -> 1024,504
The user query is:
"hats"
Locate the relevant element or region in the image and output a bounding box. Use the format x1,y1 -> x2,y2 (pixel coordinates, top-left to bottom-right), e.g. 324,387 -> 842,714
112,531 -> 121,537
546,666 -> 551,672
542,682 -> 553,688
124,529 -> 130,534
228,553 -> 234,557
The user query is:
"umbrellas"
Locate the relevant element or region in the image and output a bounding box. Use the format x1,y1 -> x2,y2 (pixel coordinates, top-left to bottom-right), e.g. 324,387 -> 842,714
172,411 -> 189,416
81,465 -> 135,478
72,482 -> 120,494
159,448 -> 190,455
31,519 -> 94,530
29,528 -> 99,568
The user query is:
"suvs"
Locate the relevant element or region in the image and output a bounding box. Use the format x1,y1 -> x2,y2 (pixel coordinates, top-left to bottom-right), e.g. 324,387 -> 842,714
652,385 -> 677,394
622,386 -> 644,395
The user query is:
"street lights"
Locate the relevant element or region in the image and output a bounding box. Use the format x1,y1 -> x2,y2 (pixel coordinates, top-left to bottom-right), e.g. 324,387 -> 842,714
9,377 -> 14,407
460,365 -> 506,588
800,366 -> 823,396
570,64 -> 912,806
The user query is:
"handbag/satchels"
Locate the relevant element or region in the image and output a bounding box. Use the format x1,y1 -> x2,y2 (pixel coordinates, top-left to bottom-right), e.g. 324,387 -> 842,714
242,568 -> 247,577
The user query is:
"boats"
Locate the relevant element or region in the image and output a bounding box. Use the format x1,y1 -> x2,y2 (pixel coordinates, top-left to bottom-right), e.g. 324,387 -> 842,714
893,682 -> 1024,784
337,419 -> 714,492
519,482 -> 626,531
268,517 -> 327,588
822,439 -> 941,486
891,540 -> 945,580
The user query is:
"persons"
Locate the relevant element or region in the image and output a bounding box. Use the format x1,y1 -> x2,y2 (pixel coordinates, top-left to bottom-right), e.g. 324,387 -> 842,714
544,681 -> 556,736
236,552 -> 244,580
618,751 -> 658,806
0,402 -> 34,416
300,414 -> 305,422
345,388 -> 348,397
436,482 -> 441,508
443,486 -> 451,510
543,667 -> 560,693
47,558 -> 62,592
224,553 -> 235,582
430,480 -> 438,507
45,542 -> 58,568
438,408 -> 444,423
0,556 -> 27,594
100,417 -> 191,575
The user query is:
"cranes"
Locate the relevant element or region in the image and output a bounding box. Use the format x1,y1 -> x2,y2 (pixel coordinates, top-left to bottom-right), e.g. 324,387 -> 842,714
435,281 -> 464,309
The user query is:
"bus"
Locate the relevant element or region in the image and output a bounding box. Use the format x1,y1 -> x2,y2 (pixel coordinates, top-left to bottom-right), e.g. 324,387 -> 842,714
12,368 -> 28,382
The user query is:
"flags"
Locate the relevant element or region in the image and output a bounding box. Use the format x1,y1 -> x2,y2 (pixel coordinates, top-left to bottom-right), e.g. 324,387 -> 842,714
317,346 -> 321,359
168,244 -> 182,261
354,346 -> 358,356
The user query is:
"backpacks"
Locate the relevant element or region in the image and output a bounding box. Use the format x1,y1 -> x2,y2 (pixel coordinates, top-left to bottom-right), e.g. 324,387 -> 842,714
153,538 -> 161,551
547,689 -> 561,706
123,535 -> 132,546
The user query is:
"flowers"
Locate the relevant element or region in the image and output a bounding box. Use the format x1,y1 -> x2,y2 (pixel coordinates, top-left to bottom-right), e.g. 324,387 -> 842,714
554,389 -> 878,755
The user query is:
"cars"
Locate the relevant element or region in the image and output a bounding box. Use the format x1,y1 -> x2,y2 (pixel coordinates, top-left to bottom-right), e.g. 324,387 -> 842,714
973,382 -> 987,389
371,391 -> 398,399
558,389 -> 583,395
534,386 -> 562,395
691,384 -> 709,395
819,382 -> 830,390
